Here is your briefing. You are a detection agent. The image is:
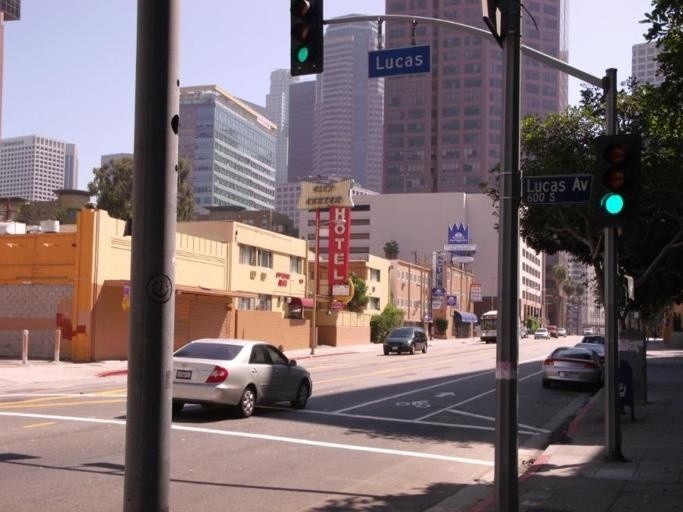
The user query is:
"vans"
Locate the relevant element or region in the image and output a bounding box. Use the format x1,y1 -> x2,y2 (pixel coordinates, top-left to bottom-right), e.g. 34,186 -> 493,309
383,326 -> 427,354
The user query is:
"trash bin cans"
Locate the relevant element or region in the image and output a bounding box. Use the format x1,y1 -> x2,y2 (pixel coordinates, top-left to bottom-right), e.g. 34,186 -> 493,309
617,360 -> 635,422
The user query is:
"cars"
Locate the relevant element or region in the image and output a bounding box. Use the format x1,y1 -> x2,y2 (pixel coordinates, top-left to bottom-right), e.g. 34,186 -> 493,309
172,328 -> 312,418
534,326 -> 567,340
542,334 -> 606,394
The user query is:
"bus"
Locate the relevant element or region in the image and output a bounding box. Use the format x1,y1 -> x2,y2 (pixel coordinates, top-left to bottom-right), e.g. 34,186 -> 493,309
481,310 -> 521,344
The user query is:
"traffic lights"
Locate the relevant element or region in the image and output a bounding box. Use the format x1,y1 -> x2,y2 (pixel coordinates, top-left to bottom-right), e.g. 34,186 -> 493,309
291,0 -> 324,77
593,133 -> 638,228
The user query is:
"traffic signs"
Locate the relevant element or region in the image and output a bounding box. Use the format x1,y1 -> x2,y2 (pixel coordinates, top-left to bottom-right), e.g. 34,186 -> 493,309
523,173 -> 594,206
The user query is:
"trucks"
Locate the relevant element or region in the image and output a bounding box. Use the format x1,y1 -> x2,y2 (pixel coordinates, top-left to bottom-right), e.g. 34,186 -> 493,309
584,328 -> 596,336
